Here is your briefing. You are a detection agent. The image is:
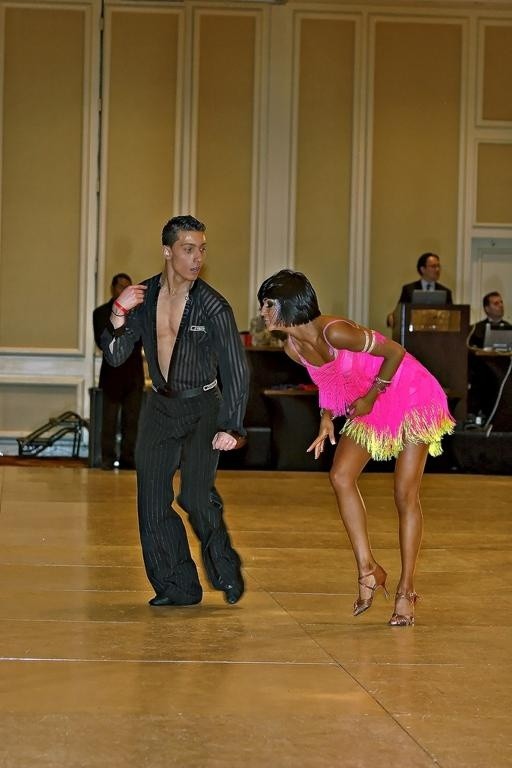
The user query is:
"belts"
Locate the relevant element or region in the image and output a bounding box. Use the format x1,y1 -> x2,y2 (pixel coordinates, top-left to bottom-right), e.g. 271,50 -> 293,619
151,378 -> 218,400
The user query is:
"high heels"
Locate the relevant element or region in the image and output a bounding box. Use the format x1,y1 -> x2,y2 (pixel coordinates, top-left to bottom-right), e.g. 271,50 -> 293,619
352,564 -> 390,617
389,587 -> 417,626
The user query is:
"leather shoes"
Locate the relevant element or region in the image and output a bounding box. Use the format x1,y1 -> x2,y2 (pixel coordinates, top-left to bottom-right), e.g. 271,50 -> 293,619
223,559 -> 244,605
148,595 -> 171,606
100,452 -> 136,471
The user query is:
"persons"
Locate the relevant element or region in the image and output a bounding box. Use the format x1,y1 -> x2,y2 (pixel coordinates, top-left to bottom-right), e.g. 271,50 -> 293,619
100,216 -> 250,607
257,269 -> 458,626
386,252 -> 453,329
465,291 -> 512,432
93,273 -> 143,471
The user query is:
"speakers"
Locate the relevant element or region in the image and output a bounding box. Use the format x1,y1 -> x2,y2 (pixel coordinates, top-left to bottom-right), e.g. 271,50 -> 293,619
89,387 -> 148,468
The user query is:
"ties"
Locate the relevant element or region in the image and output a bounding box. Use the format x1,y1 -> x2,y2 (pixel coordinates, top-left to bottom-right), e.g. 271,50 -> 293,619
426,284 -> 431,291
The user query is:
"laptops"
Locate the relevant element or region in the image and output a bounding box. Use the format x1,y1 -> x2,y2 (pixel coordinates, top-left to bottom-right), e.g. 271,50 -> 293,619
412,290 -> 447,306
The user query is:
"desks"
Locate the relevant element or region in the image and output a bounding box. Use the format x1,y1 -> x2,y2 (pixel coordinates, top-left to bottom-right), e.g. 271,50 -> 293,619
464,345 -> 512,437
257,382 -> 330,469
229,339 -> 313,427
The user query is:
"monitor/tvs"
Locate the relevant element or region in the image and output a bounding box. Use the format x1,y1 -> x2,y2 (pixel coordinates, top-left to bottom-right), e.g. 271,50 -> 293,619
485,323 -> 512,351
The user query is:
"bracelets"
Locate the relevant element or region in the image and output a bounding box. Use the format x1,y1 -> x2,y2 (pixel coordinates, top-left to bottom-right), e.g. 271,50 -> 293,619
113,300 -> 129,314
372,374 -> 392,394
319,408 -> 334,420
111,308 -> 126,318
